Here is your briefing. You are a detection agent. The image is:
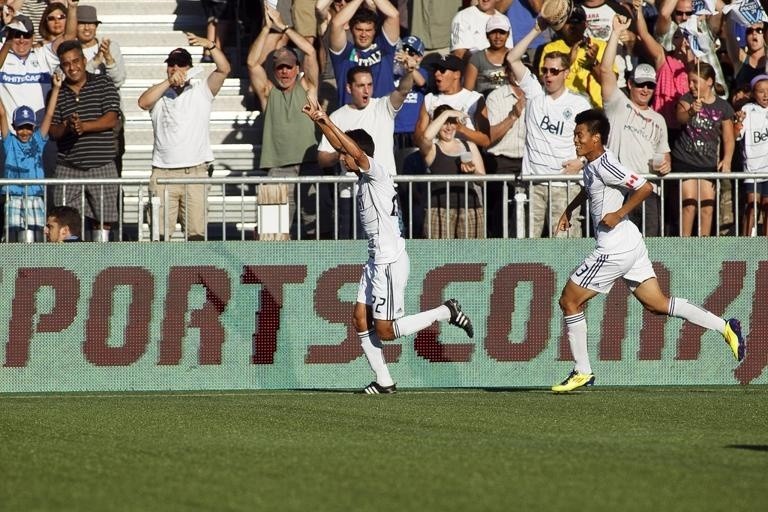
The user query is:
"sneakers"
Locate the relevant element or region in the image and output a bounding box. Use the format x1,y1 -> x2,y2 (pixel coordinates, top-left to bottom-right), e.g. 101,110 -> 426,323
721,317 -> 746,361
354,380 -> 398,395
550,370 -> 595,393
442,298 -> 475,339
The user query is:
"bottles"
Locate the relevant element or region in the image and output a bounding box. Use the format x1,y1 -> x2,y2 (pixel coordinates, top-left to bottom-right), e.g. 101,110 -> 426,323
141,224 -> 152,242
170,223 -> 185,242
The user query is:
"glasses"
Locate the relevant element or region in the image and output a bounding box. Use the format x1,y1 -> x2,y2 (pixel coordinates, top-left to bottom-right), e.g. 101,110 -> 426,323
745,27 -> 763,33
433,67 -> 450,74
632,82 -> 655,89
47,14 -> 65,21
674,9 -> 694,16
11,32 -> 33,39
541,67 -> 567,76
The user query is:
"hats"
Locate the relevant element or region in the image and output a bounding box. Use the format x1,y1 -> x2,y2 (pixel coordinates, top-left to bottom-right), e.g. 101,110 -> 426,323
429,54 -> 464,72
629,63 -> 657,85
164,47 -> 192,65
13,105 -> 36,126
691,0 -> 712,16
402,36 -> 424,57
272,46 -> 297,69
74,5 -> 101,24
484,14 -> 510,34
541,0 -> 574,31
8,14 -> 34,32
750,74 -> 768,88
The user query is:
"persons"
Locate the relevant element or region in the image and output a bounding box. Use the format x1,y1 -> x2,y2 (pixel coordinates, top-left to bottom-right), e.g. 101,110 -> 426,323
0,71 -> 65,230
66,6 -> 128,92
198,0 -> 238,63
0,2 -> 87,210
547,107 -> 746,396
43,42 -> 126,242
135,31 -> 231,242
0,0 -> 82,42
41,204 -> 85,241
37,5 -> 70,43
302,95 -> 474,396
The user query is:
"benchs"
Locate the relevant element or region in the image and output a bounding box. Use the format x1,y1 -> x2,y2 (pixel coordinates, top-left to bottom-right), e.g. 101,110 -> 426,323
0,0 -> 341,244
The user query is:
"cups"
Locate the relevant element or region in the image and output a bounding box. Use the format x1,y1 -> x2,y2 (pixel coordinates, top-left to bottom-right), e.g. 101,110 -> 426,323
92,225 -> 110,242
17,229 -> 34,244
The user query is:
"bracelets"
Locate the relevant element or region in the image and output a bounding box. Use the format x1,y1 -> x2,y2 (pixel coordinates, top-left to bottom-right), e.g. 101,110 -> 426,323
207,43 -> 219,52
